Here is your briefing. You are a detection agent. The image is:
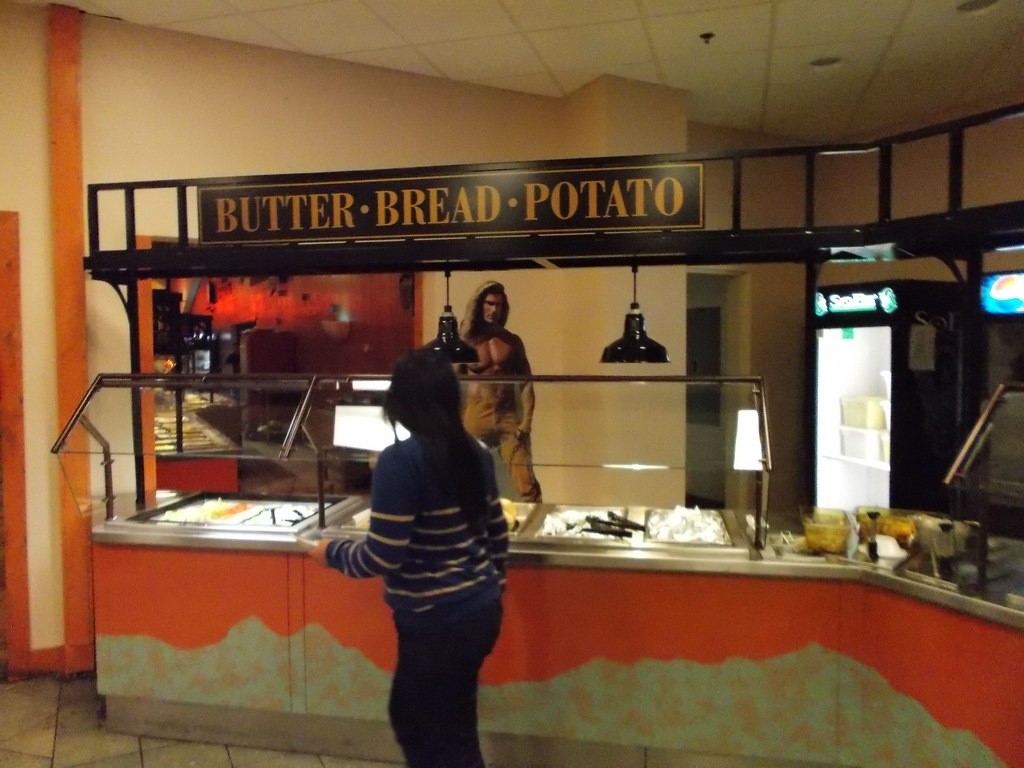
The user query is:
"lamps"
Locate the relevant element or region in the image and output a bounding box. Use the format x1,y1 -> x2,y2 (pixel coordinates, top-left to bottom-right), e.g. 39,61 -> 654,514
423,268 -> 483,365
600,265 -> 670,364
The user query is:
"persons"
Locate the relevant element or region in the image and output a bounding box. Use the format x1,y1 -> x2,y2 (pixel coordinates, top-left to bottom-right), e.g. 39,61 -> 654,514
450,279 -> 543,502
309,348 -> 510,768
